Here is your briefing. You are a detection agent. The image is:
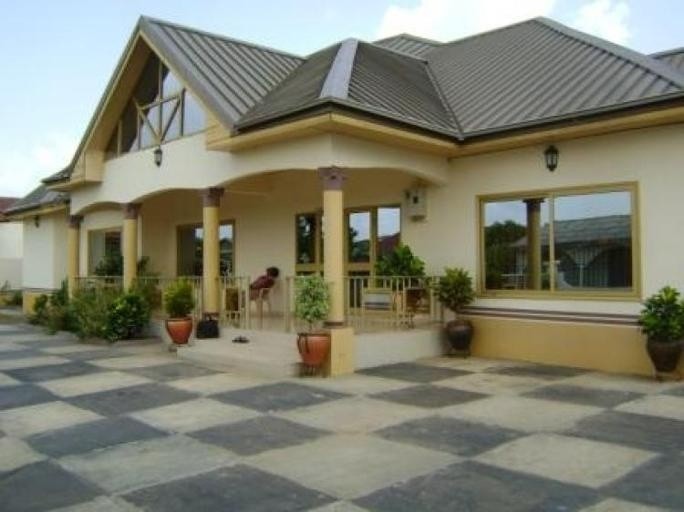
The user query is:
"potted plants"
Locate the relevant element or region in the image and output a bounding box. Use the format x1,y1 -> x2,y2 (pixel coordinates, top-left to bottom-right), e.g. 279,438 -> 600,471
373,242 -> 476,351
290,272 -> 330,367
160,276 -> 196,345
636,287 -> 683,374
100,295 -> 152,340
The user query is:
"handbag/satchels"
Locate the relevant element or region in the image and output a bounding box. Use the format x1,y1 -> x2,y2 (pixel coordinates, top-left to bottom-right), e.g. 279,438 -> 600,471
196,320 -> 217,339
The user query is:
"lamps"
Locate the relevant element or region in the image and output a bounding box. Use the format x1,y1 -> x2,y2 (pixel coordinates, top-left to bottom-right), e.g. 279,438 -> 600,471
544,146 -> 560,171
154,146 -> 163,165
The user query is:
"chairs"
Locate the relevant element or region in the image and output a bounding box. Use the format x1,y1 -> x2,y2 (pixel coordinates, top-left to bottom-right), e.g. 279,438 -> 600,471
249,276 -> 281,329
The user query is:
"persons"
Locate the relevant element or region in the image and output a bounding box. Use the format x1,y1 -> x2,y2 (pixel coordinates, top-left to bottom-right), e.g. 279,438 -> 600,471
226,268 -> 279,318
